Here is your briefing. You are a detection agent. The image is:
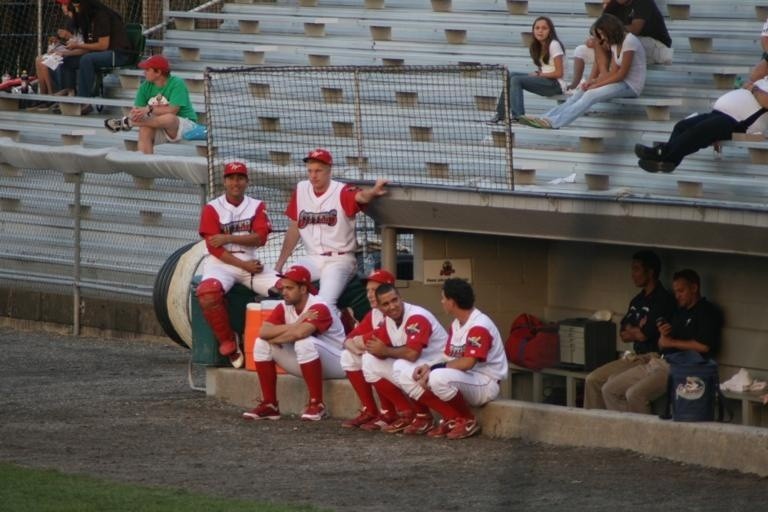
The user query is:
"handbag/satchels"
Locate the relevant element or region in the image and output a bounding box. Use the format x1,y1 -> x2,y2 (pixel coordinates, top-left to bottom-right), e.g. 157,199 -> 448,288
505,312 -> 559,372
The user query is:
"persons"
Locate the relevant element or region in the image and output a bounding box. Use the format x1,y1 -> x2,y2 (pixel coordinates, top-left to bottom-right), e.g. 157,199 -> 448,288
368,284 -> 447,437
107,53 -> 197,151
275,148 -> 387,329
36,0 -> 127,114
394,279 -> 512,440
241,266 -> 346,421
606,269 -> 727,416
196,163 -> 280,370
585,251 -> 671,407
342,271 -> 396,432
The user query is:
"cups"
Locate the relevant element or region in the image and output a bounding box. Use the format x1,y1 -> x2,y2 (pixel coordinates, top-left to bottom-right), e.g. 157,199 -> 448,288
2,73 -> 11,83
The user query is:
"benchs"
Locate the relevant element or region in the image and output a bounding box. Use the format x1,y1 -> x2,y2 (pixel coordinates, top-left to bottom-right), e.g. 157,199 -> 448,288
0,180 -> 413,304
0,0 -> 768,207
497,356 -> 768,429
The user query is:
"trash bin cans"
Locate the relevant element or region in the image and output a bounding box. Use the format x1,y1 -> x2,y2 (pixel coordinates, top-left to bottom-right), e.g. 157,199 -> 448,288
189,275 -> 256,389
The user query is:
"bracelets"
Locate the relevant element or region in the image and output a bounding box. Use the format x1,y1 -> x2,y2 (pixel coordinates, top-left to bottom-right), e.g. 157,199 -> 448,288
430,363 -> 444,370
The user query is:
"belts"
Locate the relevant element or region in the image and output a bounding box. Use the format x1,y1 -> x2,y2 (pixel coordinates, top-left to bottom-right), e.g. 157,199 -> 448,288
320,251 -> 344,256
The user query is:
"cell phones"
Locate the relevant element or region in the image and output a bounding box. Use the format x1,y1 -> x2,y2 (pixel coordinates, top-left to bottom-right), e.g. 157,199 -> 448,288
656,316 -> 667,325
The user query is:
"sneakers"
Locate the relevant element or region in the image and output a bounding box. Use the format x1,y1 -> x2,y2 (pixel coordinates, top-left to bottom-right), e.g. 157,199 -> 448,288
486,112 -> 552,129
634,142 -> 676,173
241,399 -> 281,421
104,116 -> 132,132
25,100 -> 94,116
341,406 -> 481,441
227,331 -> 244,369
300,397 -> 328,422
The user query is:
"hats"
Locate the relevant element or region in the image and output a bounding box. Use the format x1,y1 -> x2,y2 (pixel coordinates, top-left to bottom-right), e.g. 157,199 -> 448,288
276,265 -> 312,286
361,269 -> 396,287
303,148 -> 333,167
223,161 -> 248,178
137,54 -> 170,72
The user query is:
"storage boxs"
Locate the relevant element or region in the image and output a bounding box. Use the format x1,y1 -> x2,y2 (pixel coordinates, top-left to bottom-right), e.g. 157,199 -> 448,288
555,316 -> 617,371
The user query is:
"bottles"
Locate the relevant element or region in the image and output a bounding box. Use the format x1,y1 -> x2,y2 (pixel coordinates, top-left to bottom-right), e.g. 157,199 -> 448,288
733,75 -> 745,91
19,70 -> 29,94
